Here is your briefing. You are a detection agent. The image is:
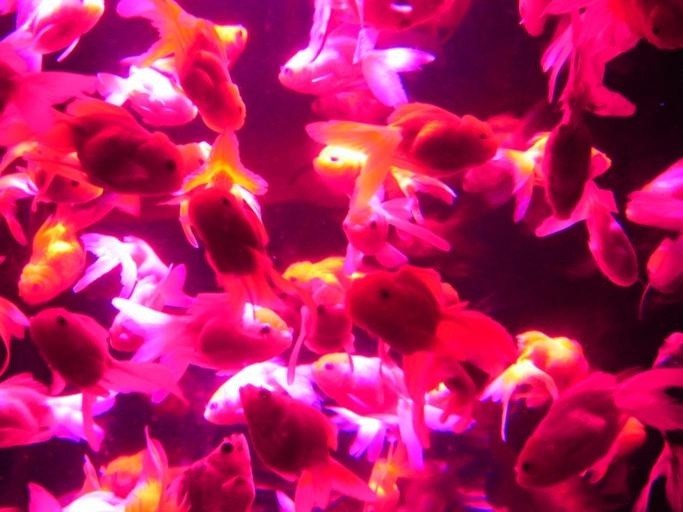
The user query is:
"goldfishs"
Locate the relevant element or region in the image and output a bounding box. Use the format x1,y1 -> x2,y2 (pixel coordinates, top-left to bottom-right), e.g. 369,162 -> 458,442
0,0 -> 683,512
